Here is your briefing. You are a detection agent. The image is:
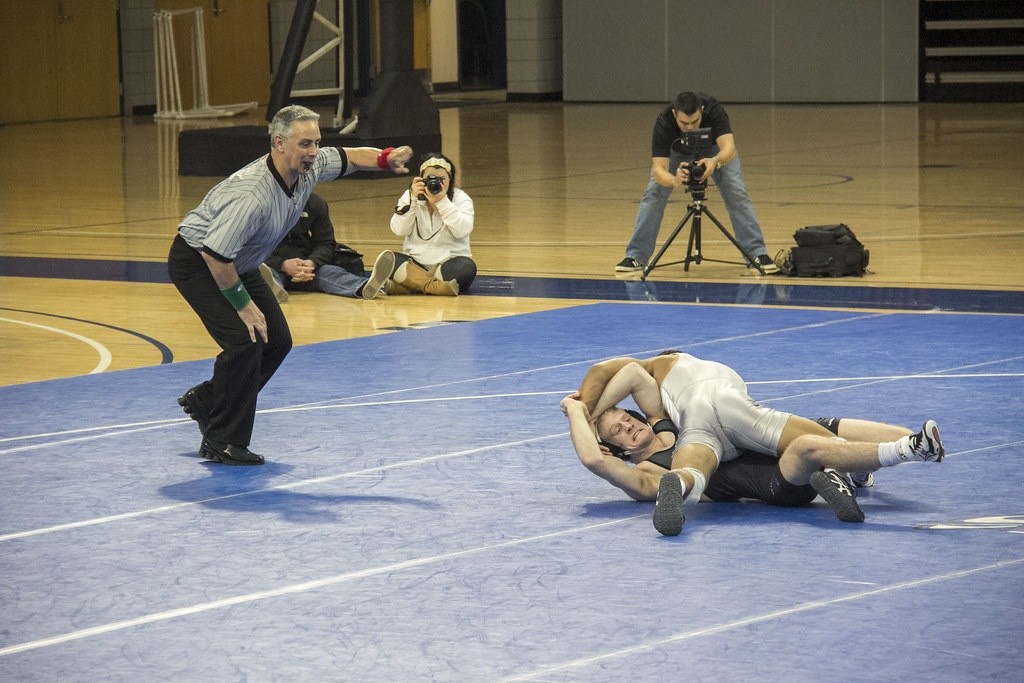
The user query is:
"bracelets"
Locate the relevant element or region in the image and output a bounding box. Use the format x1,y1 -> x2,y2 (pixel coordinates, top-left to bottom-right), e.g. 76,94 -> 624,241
219,278 -> 251,311
377,147 -> 393,169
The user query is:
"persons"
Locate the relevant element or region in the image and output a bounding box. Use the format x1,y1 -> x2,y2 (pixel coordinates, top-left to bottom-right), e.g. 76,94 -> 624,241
615,92 -> 781,274
561,390 -> 944,508
385,152 -> 477,295
264,192 -> 396,299
167,104 -> 414,464
579,350 -> 874,537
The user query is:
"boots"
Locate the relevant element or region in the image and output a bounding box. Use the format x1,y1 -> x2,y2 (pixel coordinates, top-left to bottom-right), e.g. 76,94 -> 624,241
386,260 -> 461,295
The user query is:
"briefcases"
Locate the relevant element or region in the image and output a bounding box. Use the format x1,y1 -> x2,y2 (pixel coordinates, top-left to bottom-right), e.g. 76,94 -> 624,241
791,243 -> 866,278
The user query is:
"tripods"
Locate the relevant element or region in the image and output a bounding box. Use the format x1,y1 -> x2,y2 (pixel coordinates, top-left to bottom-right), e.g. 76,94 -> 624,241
641,191 -> 766,281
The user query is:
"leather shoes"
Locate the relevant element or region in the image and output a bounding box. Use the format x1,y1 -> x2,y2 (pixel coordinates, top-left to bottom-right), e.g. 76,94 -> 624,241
198,435 -> 265,466
177,389 -> 209,434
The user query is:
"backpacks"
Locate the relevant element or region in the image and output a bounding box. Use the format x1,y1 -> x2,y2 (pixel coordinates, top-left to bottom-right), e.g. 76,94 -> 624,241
794,224 -> 861,247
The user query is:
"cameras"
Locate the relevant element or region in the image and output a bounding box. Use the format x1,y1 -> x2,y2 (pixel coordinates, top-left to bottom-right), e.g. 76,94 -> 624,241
417,175 -> 443,200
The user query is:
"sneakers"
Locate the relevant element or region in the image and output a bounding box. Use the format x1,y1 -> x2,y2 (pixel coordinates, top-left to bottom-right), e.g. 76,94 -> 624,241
809,468 -> 866,524
653,472 -> 686,537
909,420 -> 946,463
847,473 -> 873,488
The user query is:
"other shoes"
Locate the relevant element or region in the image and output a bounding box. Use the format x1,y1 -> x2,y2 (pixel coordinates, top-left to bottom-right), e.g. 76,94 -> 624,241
748,255 -> 780,273
362,249 -> 396,300
257,263 -> 288,305
615,257 -> 644,272
